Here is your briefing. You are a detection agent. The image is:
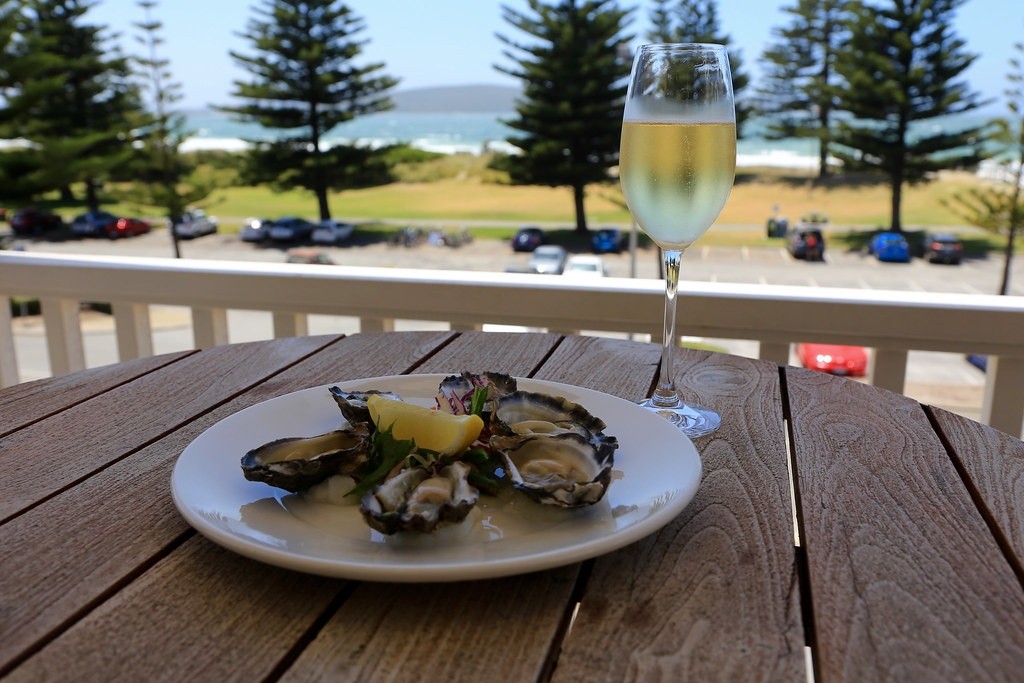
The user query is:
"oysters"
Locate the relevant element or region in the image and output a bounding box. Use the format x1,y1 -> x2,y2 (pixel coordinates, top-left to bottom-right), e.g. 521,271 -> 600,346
240,371 -> 621,537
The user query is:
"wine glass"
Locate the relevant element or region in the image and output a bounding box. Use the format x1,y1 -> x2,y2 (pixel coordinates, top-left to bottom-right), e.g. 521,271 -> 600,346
619,44 -> 737,439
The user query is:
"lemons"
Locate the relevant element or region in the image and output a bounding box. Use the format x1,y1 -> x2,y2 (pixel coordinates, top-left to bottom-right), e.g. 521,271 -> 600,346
366,393 -> 484,458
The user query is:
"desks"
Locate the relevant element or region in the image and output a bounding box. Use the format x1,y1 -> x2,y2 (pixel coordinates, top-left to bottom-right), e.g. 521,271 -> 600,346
0,329 -> 1024,683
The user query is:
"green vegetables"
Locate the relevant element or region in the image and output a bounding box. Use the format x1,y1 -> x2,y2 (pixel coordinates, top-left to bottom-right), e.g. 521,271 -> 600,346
341,384 -> 501,497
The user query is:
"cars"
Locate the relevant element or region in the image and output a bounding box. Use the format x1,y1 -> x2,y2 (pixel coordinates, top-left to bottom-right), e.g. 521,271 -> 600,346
70,212 -> 150,240
513,229 -> 544,250
870,233 -> 909,263
795,343 -> 868,378
922,234 -> 963,264
242,214 -> 356,245
593,228 -> 621,252
788,228 -> 824,258
532,244 -> 567,275
561,254 -> 603,276
171,211 -> 216,238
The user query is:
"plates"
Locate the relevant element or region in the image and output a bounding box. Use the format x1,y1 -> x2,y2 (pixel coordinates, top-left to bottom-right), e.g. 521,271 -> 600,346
170,374 -> 703,582
